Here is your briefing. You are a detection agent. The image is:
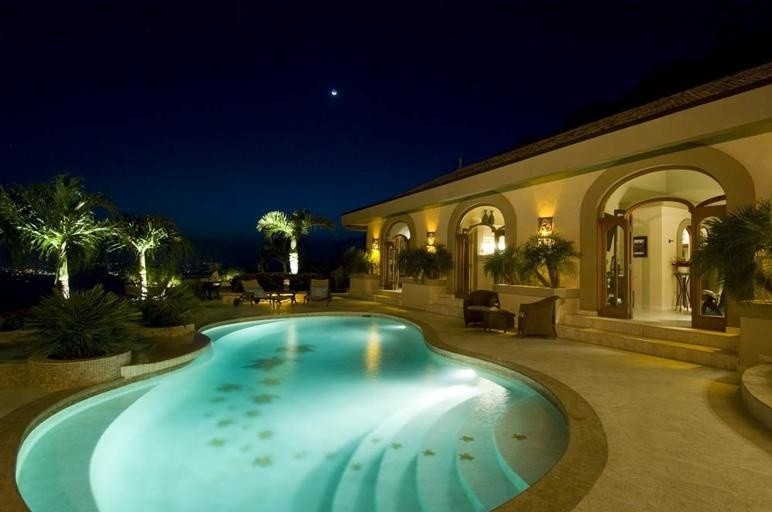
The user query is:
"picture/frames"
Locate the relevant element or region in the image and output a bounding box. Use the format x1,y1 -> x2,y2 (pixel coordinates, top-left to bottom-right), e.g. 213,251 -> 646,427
633,236 -> 647,257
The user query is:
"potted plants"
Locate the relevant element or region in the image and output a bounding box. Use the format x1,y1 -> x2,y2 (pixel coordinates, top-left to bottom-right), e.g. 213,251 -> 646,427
671,257 -> 689,273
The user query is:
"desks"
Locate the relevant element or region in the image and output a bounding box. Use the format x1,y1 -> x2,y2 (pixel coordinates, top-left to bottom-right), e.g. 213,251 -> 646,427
484,309 -> 516,333
674,273 -> 691,313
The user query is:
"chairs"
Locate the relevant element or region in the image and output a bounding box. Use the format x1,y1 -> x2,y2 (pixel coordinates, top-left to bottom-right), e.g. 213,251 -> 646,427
515,296 -> 560,338
239,278 -> 298,306
463,290 -> 500,327
302,278 -> 333,307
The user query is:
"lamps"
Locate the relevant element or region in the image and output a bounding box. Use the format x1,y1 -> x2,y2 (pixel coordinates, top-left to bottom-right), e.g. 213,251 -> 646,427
426,232 -> 437,247
371,238 -> 380,250
537,217 -> 553,238
